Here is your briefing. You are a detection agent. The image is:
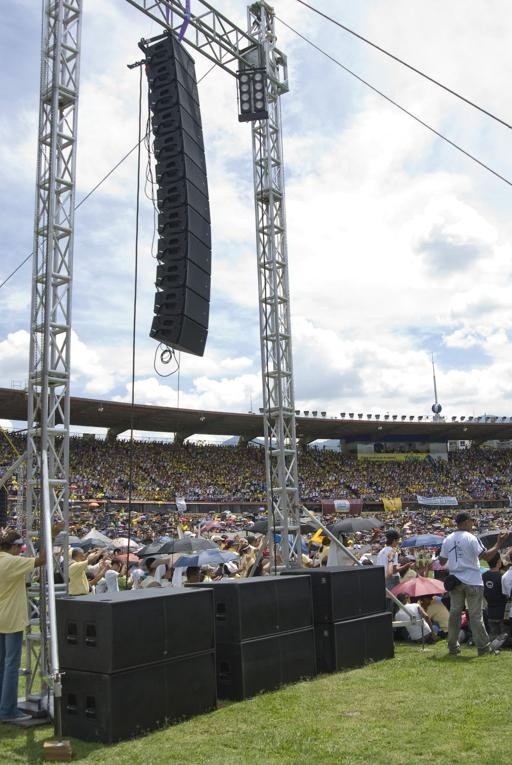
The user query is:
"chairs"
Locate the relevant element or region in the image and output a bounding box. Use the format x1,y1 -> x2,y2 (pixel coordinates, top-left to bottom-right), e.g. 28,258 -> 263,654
492,632 -> 509,648
1,711 -> 34,724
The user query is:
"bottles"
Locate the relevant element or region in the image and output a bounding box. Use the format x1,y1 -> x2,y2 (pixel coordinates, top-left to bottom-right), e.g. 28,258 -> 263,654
455,512 -> 477,524
385,529 -> 405,539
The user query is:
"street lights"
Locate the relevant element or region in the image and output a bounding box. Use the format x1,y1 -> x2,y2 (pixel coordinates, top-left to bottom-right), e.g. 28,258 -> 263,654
444,575 -> 461,592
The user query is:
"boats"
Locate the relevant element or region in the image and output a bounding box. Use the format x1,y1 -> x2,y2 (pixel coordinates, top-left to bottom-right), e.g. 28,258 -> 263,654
52,650 -> 219,746
145,34 -> 210,357
184,574 -> 313,645
57,587 -> 216,675
318,611 -> 395,671
220,626 -> 318,702
278,565 -> 387,624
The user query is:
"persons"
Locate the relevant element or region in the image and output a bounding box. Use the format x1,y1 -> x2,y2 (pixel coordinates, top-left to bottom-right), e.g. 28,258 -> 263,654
0,518 -> 67,725
0,506 -> 296,616
379,530 -> 412,616
459,549 -> 512,649
396,592 -> 451,642
0,430 -> 293,501
303,508 -> 512,576
439,514 -> 509,654
297,445 -> 512,502
